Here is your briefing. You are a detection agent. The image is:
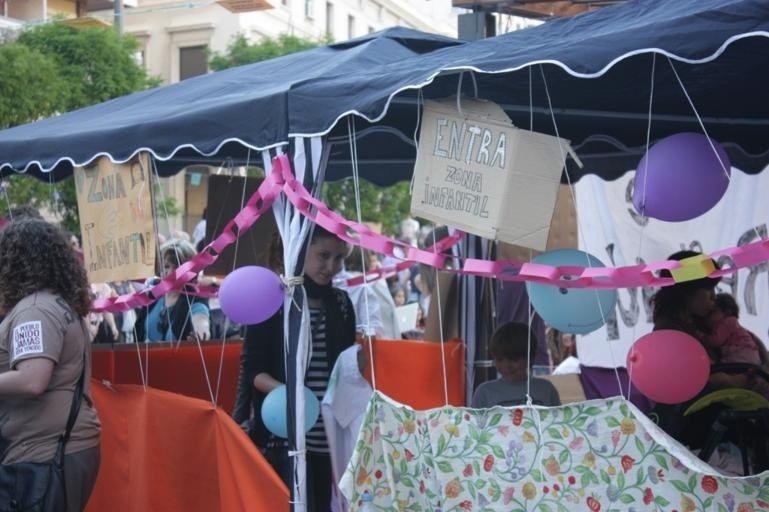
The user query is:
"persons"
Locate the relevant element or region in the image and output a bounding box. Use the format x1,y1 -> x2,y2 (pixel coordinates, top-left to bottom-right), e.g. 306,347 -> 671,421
0,206 -> 452,512
471,320 -> 561,408
649,251 -> 769,477
698,290 -> 768,393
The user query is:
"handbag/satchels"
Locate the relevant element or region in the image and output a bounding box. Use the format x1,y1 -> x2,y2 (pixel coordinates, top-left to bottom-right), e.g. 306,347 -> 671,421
2,453 -> 71,510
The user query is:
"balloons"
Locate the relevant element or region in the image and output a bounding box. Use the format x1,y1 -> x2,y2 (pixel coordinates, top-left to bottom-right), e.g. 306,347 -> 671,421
625,329 -> 713,406
522,248 -> 618,336
631,131 -> 731,222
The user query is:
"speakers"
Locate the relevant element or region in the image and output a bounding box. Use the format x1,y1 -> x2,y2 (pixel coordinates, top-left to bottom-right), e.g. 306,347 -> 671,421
458,13 -> 496,41
